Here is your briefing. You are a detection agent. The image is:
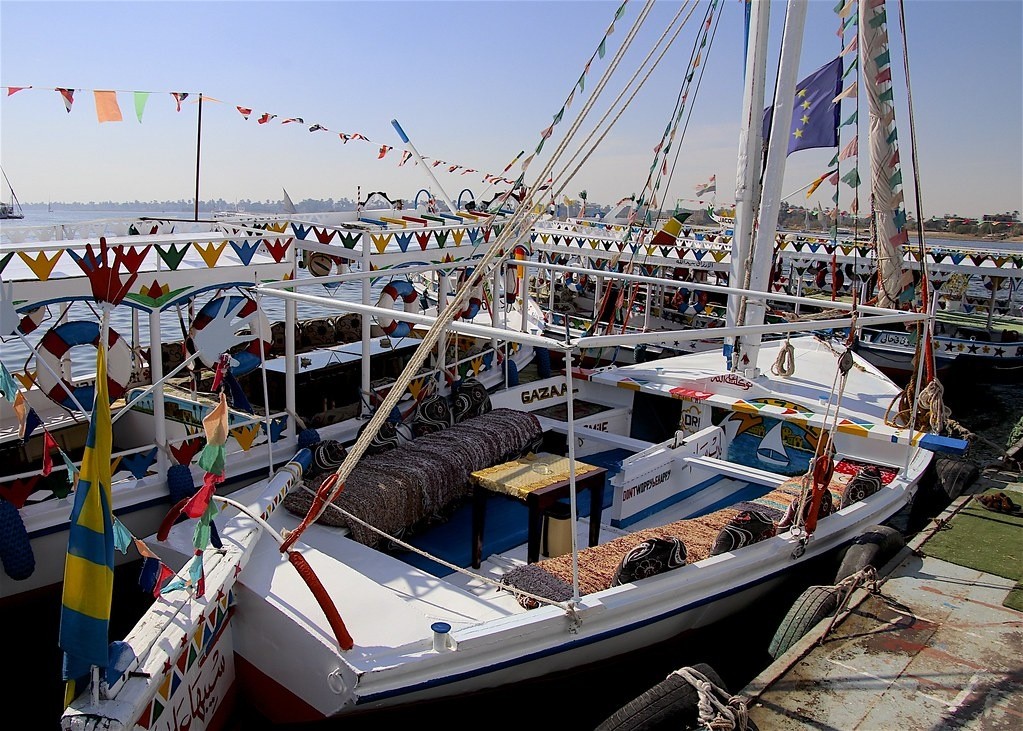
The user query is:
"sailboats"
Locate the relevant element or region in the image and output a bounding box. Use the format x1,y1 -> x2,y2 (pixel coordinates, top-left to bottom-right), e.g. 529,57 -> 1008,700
135,0 -> 975,730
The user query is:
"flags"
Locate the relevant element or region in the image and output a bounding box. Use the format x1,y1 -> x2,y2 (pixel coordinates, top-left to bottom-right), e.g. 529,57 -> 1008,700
237,107 -> 328,132
833,0 -> 859,102
806,169 -> 838,197
339,133 -> 413,166
695,177 -> 716,197
649,213 -> 691,245
431,159 -> 477,175
482,175 -> 515,185
8,87 -> 189,123
828,111 -> 861,239
58,348 -> 114,708
760,57 -> 843,184
875,68 -> 895,126
869,0 -> 890,67
887,128 -> 909,247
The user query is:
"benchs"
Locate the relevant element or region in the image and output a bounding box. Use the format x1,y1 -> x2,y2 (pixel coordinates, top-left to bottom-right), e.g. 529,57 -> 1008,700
497,476 -> 849,610
0,402 -> 127,465
285,408 -> 546,547
126,338 -> 362,428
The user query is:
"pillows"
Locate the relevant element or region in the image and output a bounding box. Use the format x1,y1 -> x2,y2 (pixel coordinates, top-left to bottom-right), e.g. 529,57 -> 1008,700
607,535 -> 688,588
708,510 -> 777,558
145,312 -> 362,376
359,340 -> 505,410
839,465 -> 882,510
776,489 -> 832,536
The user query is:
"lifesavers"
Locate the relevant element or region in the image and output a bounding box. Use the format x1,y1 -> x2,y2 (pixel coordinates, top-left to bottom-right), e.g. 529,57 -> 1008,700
676,287 -> 707,315
188,296 -> 272,378
816,266 -> 844,292
565,263 -> 588,292
833,524 -> 906,585
844,264 -> 865,281
594,663 -> 729,731
36,321 -> 132,412
378,280 -> 419,338
907,458 -> 980,543
322,255 -> 346,288
805,260 -> 827,274
557,253 -> 570,266
981,275 -> 1008,291
767,585 -> 845,661
456,267 -> 484,320
506,264 -> 517,303
11,306 -> 46,336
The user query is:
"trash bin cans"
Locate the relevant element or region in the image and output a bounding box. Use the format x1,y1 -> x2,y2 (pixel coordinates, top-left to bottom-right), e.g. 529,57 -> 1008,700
540,502 -> 581,559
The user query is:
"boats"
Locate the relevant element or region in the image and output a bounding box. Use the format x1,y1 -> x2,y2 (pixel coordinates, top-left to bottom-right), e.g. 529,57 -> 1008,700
527,215 -> 1023,387
0,210 -> 554,608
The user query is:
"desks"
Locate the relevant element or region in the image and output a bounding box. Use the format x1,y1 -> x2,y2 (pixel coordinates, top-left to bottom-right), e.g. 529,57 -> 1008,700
470,451 -> 609,570
258,335 -> 422,414
